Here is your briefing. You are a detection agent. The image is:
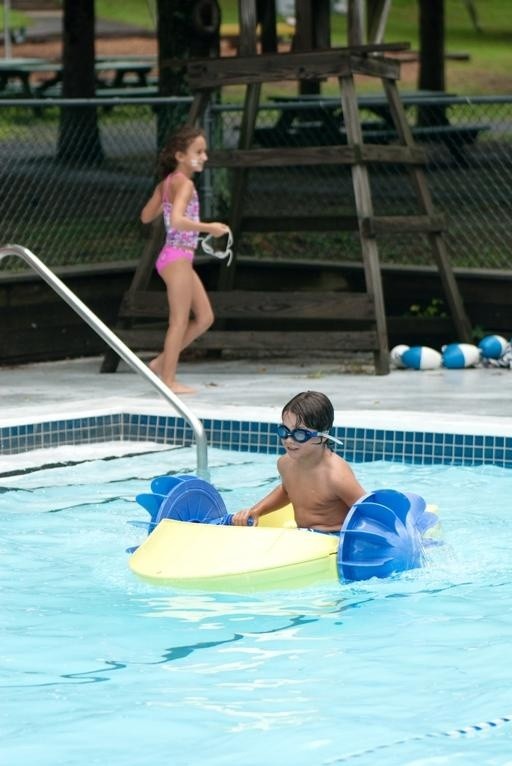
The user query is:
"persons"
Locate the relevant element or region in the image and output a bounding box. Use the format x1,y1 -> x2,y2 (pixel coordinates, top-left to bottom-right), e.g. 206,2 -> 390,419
232,391 -> 367,533
140,125 -> 216,392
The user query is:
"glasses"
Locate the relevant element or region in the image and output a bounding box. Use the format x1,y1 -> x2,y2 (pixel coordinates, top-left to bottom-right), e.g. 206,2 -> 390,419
278,425 -> 320,442
201,226 -> 233,267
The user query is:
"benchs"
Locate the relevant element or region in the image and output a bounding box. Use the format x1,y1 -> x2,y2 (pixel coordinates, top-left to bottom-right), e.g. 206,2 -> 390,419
233,93 -> 492,150
1,58 -> 161,118
